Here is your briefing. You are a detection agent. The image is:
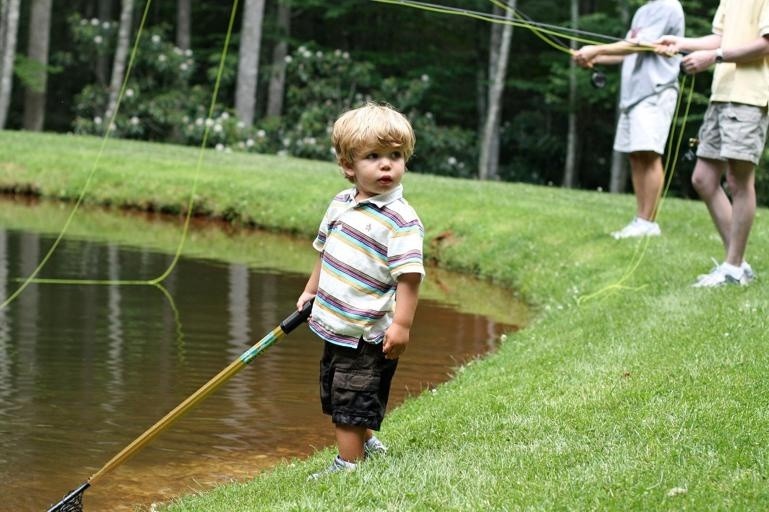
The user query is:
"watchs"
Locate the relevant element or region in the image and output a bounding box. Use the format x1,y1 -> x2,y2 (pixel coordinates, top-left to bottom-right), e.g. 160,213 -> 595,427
714,48 -> 723,64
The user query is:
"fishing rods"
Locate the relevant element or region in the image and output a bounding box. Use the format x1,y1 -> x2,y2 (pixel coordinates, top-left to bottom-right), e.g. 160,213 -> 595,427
491,1 -> 609,87
375,1 -> 688,59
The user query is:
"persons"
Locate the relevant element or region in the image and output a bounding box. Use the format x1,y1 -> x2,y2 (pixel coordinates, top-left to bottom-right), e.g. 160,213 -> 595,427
572,2 -> 686,241
649,3 -> 769,289
296,105 -> 427,484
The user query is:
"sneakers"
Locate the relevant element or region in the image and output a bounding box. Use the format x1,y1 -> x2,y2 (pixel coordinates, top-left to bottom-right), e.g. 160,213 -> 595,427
611,218 -> 661,239
307,454 -> 360,484
692,263 -> 754,290
363,440 -> 387,460
